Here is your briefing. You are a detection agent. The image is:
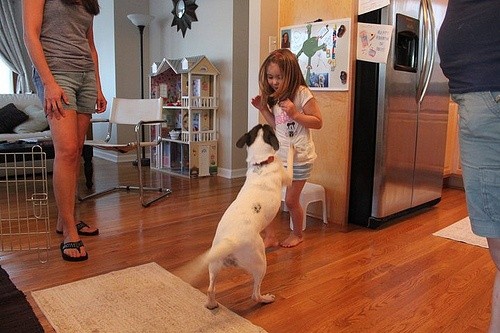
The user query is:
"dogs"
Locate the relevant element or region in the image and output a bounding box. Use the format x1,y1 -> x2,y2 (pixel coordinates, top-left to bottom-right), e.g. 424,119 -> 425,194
175,124 -> 297,310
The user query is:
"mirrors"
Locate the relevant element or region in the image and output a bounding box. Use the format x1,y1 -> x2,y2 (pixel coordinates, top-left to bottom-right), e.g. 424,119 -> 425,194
170,0 -> 199,38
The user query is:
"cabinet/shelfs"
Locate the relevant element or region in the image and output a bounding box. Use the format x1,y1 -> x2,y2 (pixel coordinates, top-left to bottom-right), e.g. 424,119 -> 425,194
441,100 -> 463,176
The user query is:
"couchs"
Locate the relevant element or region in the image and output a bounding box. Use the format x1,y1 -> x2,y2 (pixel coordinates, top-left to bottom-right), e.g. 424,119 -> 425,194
0,94 -> 96,195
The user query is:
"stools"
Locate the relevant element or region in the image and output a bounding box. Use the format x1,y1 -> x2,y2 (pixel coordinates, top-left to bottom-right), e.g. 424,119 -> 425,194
281,181 -> 328,231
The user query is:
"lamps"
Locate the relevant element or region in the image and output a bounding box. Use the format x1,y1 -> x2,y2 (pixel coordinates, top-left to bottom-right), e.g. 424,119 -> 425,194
127,14 -> 157,166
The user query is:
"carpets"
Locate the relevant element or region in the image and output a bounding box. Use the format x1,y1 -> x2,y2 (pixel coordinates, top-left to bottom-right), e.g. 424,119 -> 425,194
32,260 -> 269,333
432,210 -> 489,248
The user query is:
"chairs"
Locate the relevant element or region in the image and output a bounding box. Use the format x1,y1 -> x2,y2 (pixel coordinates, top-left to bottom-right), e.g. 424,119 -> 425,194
75,97 -> 174,207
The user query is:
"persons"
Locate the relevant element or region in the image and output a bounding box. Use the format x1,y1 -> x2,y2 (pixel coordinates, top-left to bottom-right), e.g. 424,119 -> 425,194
437,0 -> 500,333
22,0 -> 108,262
251,49 -> 322,250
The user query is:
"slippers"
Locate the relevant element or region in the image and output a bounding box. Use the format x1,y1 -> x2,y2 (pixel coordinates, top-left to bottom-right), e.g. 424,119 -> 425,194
61,240 -> 89,261
56,221 -> 99,236
